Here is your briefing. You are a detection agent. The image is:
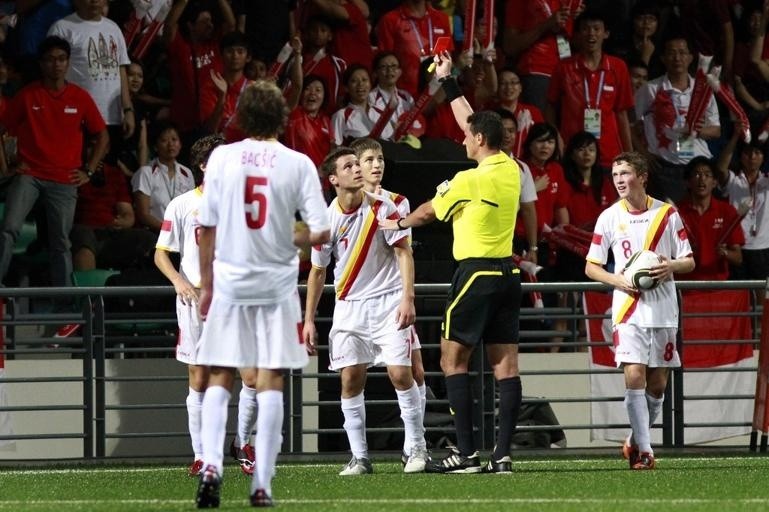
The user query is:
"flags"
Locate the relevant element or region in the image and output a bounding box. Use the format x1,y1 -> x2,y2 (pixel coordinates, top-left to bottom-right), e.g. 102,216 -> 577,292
578,286 -> 757,447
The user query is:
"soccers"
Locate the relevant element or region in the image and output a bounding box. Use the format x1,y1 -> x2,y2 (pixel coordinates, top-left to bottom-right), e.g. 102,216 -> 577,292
624,250 -> 662,293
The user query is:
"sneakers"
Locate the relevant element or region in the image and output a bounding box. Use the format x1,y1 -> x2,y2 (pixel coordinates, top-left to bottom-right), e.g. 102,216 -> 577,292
192,443 -> 275,509
404,448 -> 432,472
622,439 -> 639,468
481,455 -> 512,474
424,449 -> 481,474
338,455 -> 371,476
634,452 -> 656,469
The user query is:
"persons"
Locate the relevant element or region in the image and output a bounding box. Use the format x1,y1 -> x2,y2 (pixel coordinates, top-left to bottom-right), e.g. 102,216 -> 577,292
195,80 -> 331,509
300,146 -> 430,478
585,151 -> 697,468
1,2 -> 769,351
150,134 -> 257,477
352,135 -> 450,474
377,50 -> 523,476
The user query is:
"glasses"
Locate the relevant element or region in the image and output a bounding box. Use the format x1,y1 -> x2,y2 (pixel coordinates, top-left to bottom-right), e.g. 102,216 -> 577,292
379,64 -> 401,70
499,82 -> 521,85
